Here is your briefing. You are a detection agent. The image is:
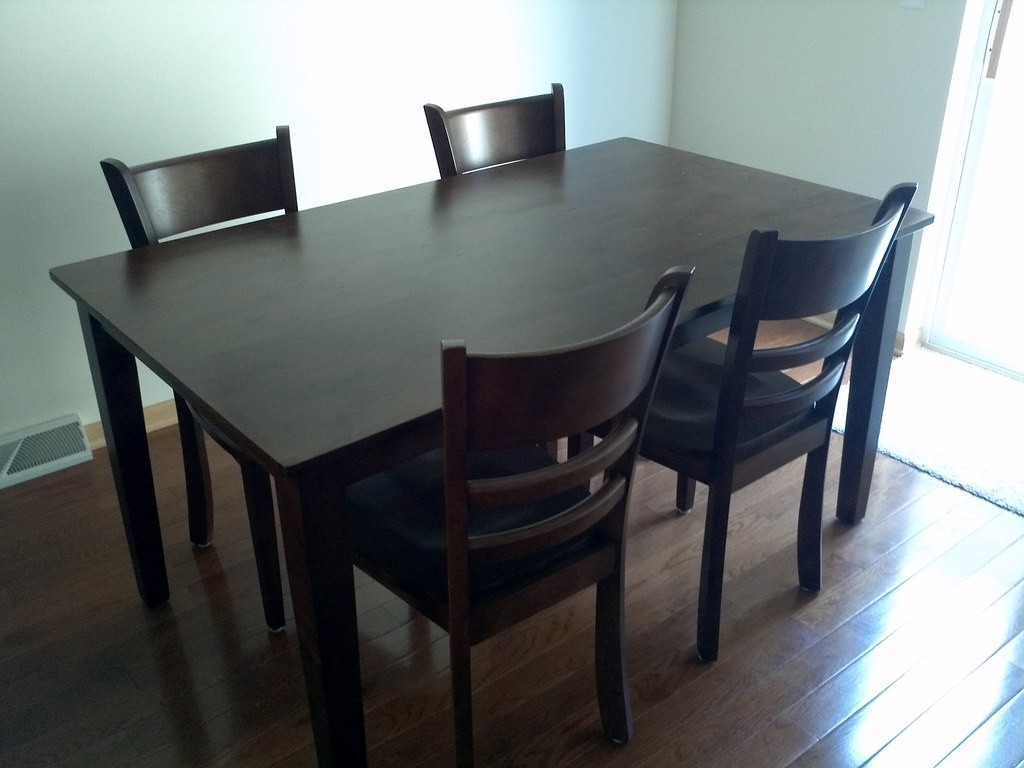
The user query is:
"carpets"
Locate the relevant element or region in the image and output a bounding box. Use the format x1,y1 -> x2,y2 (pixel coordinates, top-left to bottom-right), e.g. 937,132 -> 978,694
830,345 -> 1024,519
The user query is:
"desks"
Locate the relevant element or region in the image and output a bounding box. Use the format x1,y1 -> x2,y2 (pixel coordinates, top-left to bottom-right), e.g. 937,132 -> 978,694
50,135 -> 933,768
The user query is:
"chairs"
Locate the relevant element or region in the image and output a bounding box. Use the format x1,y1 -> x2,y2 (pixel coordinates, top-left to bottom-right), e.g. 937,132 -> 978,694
99,126 -> 300,631
349,265 -> 698,768
425,81 -> 917,660
422,75 -> 565,178
